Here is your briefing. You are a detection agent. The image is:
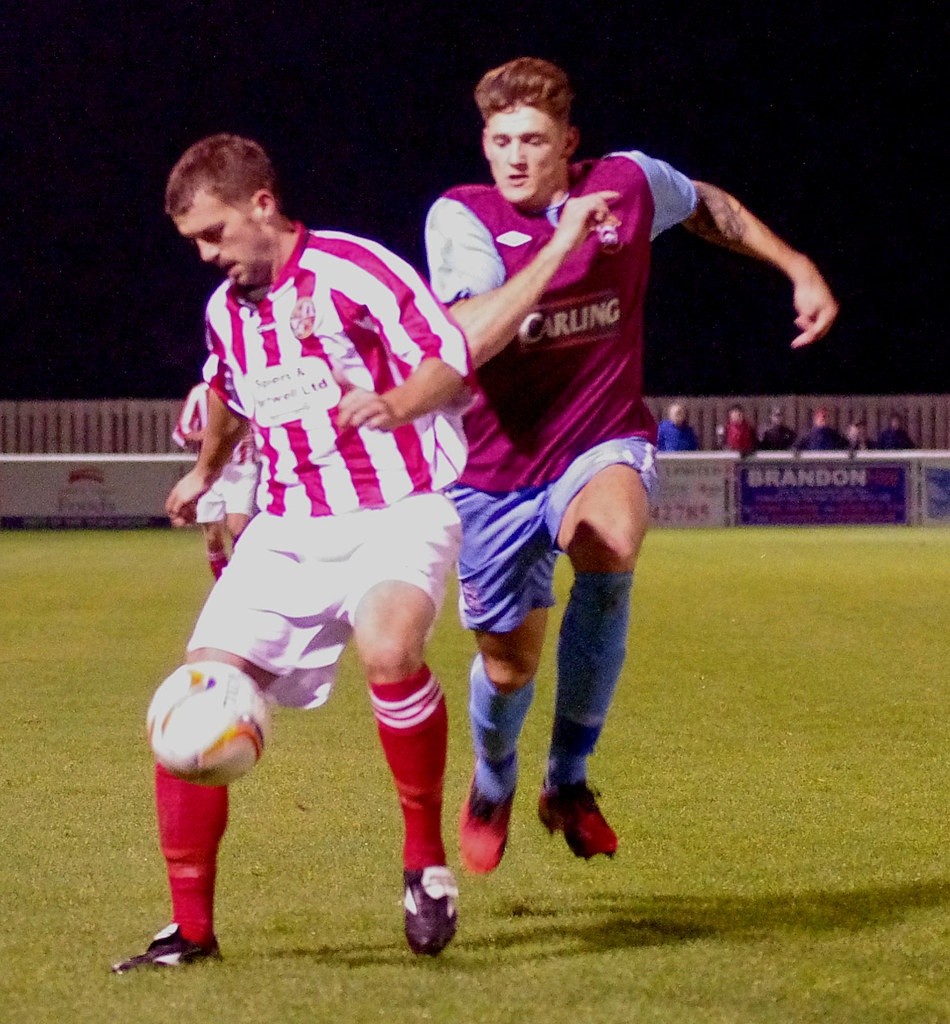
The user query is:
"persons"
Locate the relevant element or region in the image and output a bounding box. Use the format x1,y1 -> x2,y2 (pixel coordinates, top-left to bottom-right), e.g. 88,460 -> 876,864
112,137 -> 473,976
659,404 -> 700,450
858,414 -> 917,449
757,410 -> 797,451
716,405 -> 754,454
791,407 -> 859,450
425,57 -> 838,873
170,383 -> 261,578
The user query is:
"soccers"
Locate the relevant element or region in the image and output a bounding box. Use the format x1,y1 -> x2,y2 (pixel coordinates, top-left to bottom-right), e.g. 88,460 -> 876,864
145,660 -> 272,787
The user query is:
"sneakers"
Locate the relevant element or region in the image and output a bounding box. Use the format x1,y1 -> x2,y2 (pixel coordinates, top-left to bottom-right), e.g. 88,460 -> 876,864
535,779 -> 617,860
113,923 -> 221,974
460,769 -> 517,874
402,865 -> 459,958
